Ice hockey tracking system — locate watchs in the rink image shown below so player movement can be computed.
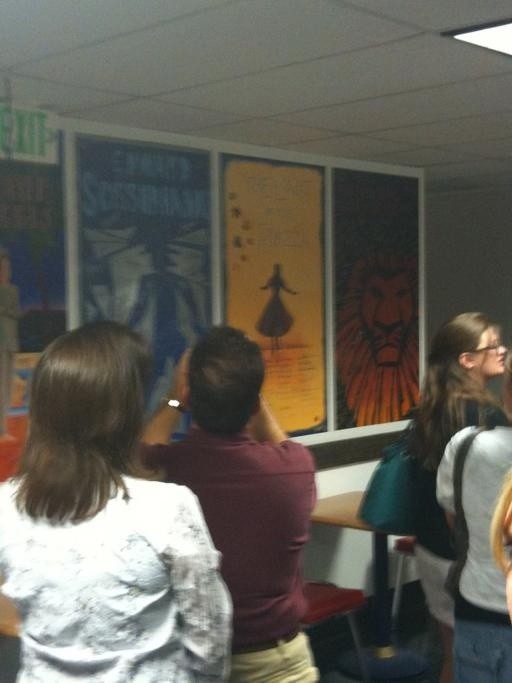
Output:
[161,394,188,413]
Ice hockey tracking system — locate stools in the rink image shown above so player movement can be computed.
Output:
[298,582,372,683]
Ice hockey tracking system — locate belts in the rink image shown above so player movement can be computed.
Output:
[231,625,300,654]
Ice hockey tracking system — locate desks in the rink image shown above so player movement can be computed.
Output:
[309,491,431,680]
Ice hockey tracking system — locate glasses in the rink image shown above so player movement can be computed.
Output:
[473,340,501,352]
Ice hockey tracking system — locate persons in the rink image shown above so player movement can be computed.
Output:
[435,344,512,682]
[133,326,325,682]
[1,319,234,683]
[0,247,48,443]
[254,260,299,355]
[404,312,511,682]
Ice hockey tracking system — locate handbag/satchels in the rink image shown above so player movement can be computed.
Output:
[356,428,422,535]
